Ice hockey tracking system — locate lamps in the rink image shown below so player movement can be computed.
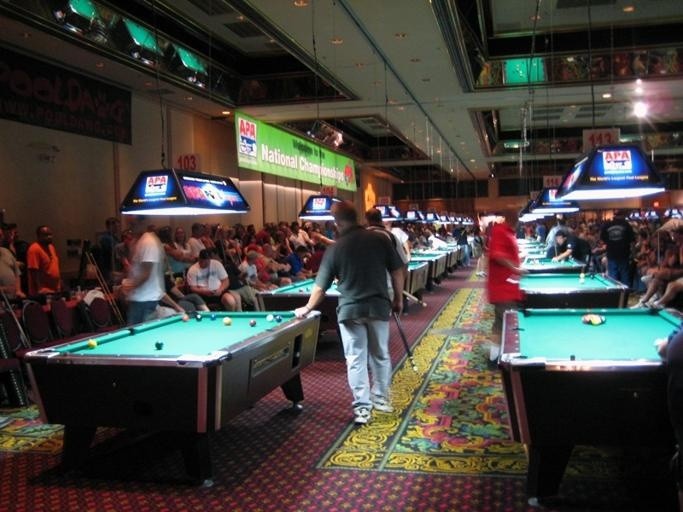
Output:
[662,206,682,220]
[451,216,477,226]
[369,56,405,222]
[442,138,451,225]
[118,0,251,216]
[427,123,440,224]
[293,1,348,221]
[553,2,667,202]
[644,208,660,221]
[625,209,642,221]
[529,56,581,216]
[516,200,544,222]
[407,98,427,224]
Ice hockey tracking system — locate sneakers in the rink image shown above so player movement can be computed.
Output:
[367,394,394,412]
[354,407,372,424]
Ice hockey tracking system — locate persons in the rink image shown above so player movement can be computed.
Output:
[26,225,62,303]
[189,222,206,258]
[156,278,212,318]
[0,222,10,248]
[274,219,338,287]
[114,214,165,326]
[157,226,200,282]
[225,222,274,312]
[486,208,530,371]
[186,250,236,312]
[543,210,683,312]
[6,223,26,264]
[146,224,159,238]
[174,227,198,272]
[79,217,122,272]
[657,314,683,386]
[517,221,545,242]
[119,230,133,269]
[293,200,404,426]
[406,221,448,250]
[0,246,26,304]
[472,230,487,278]
[210,223,223,248]
[457,225,470,268]
[202,222,215,252]
[390,221,411,287]
[365,208,404,311]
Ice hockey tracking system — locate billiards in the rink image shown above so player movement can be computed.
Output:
[580,272,586,284]
[182,314,190,322]
[590,274,595,279]
[196,314,202,320]
[306,287,311,292]
[276,315,282,322]
[541,261,545,266]
[582,313,606,326]
[87,339,96,347]
[299,288,303,292]
[224,317,231,325]
[155,341,164,349]
[210,314,216,320]
[249,320,256,326]
[267,315,273,322]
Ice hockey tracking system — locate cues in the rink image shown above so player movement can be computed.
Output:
[656,224,661,299]
[390,280,429,309]
[235,240,262,311]
[552,227,558,262]
[390,311,421,372]
[216,223,230,269]
[83,248,126,327]
